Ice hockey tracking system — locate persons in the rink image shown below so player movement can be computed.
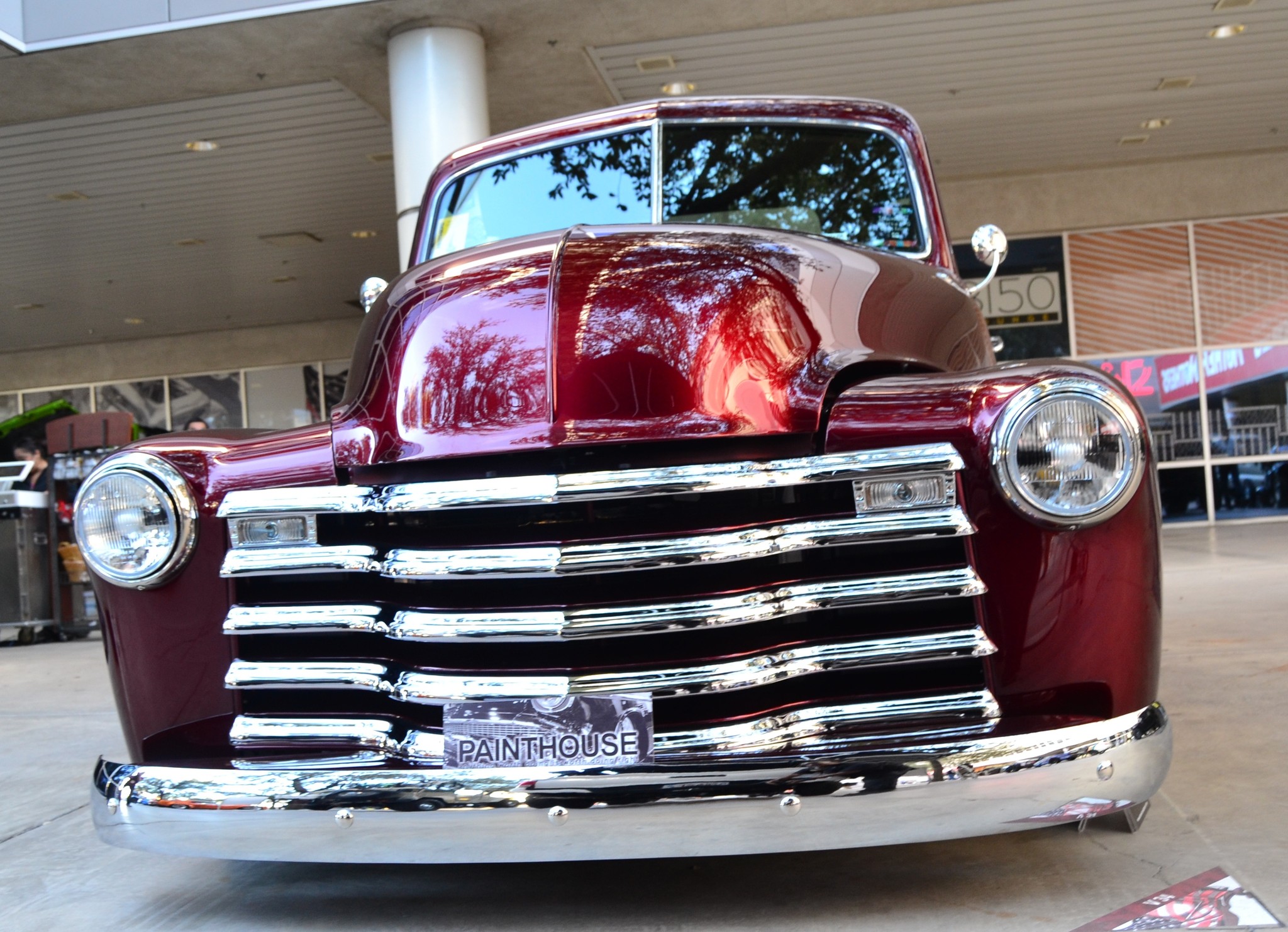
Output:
[1212,428,1247,511]
[8,438,56,494]
[184,418,210,433]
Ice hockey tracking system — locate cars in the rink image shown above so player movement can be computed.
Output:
[66,80,1169,868]
[1218,463,1267,503]
